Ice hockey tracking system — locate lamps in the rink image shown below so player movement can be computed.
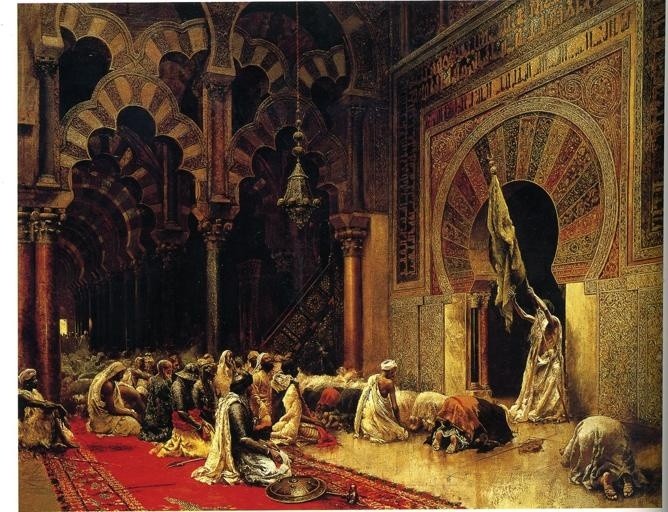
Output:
[277,2,322,230]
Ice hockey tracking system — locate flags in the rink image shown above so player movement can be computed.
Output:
[487,173,527,334]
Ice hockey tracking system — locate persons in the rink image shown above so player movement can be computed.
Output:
[508,286,569,423]
[560,414,651,501]
[17,329,511,493]
